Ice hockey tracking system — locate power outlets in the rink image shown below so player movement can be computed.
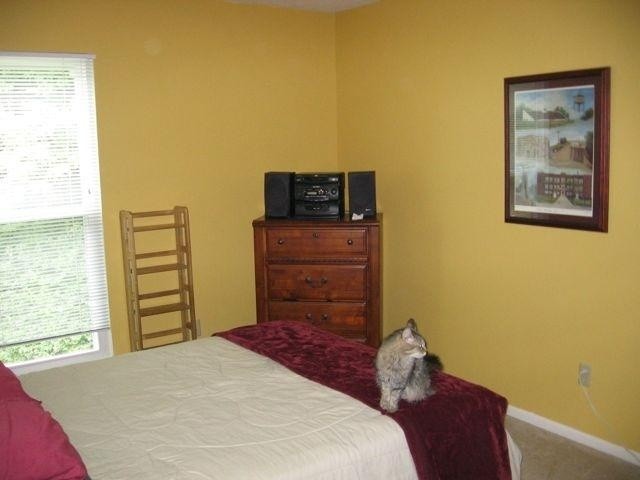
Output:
[576,361,593,388]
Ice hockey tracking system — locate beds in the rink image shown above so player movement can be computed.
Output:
[1,317,510,480]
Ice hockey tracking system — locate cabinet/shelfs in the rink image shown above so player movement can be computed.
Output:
[251,213,385,350]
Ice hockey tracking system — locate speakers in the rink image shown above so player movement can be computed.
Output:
[265,171,295,219]
[348,172,376,219]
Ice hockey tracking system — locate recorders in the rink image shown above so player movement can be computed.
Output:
[295,171,345,220]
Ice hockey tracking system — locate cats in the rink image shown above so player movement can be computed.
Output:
[373,317,443,415]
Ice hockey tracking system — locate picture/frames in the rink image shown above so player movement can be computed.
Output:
[502,66,612,234]
